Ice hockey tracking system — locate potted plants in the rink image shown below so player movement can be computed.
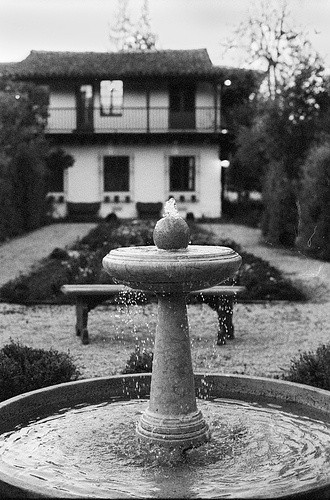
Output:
[169,195,174,200]
[114,195,120,203]
[48,195,55,204]
[59,195,64,203]
[125,195,131,203]
[191,194,197,203]
[179,196,186,202]
[104,196,110,202]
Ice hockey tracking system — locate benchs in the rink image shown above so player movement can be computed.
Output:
[59,283,248,346]
